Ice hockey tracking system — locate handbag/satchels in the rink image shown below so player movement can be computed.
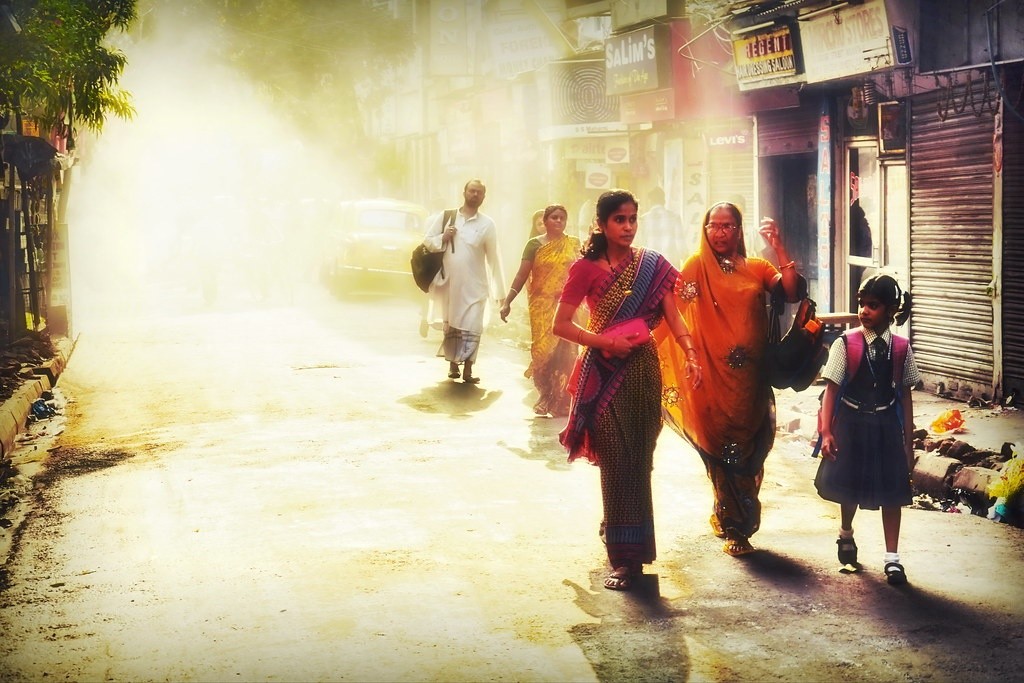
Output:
[597,317,649,359]
[411,209,457,293]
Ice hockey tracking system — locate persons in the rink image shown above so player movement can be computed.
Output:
[552,189,706,589]
[652,200,809,556]
[419,180,684,417]
[812,273,922,583]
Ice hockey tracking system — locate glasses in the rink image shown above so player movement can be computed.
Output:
[704,223,740,232]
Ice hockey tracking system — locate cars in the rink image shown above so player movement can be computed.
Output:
[315,198,431,316]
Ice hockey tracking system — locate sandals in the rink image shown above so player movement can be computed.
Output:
[710,513,727,538]
[604,570,642,590]
[723,537,754,557]
[598,520,606,544]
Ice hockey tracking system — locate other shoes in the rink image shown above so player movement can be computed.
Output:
[884,562,908,585]
[447,369,461,378]
[559,402,571,416]
[462,376,480,384]
[533,394,553,415]
[836,535,858,565]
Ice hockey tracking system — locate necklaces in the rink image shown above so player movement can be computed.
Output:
[604,247,635,295]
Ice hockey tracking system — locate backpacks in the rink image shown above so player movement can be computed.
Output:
[767,272,829,392]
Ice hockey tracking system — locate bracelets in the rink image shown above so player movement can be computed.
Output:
[778,261,795,271]
[685,348,696,356]
[510,288,518,295]
[675,334,691,342]
[578,329,583,347]
